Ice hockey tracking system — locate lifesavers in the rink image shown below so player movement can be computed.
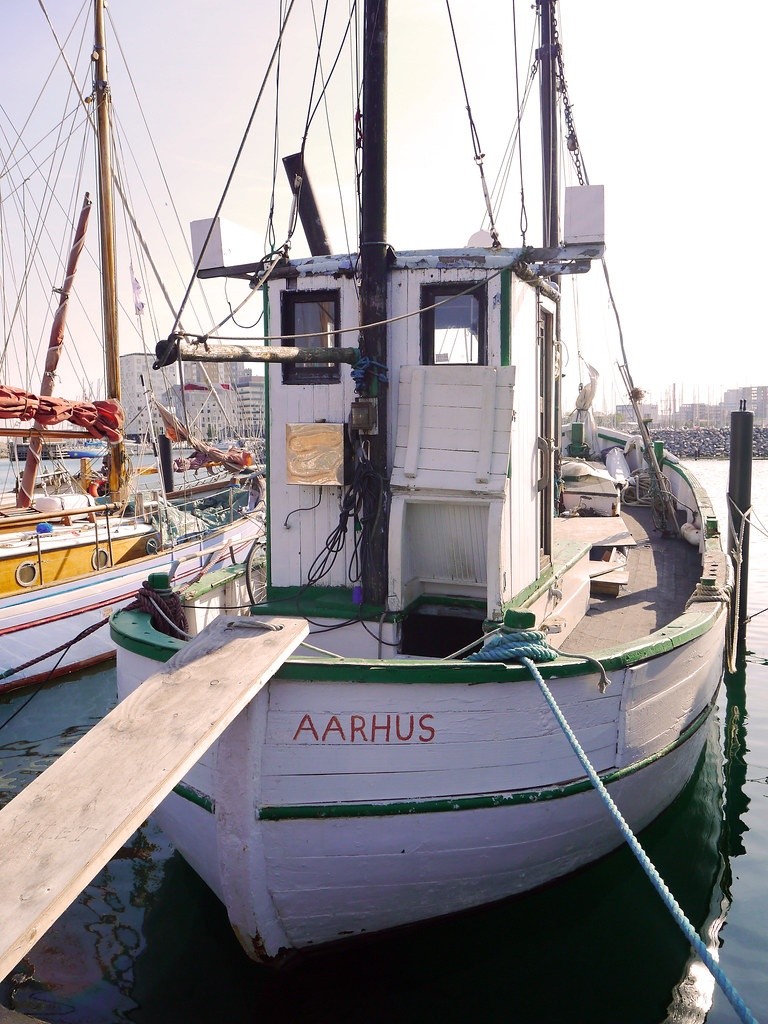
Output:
[90,479,110,497]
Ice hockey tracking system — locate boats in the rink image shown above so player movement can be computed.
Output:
[65,437,106,457]
[0,430,269,689]
[109,237,723,955]
